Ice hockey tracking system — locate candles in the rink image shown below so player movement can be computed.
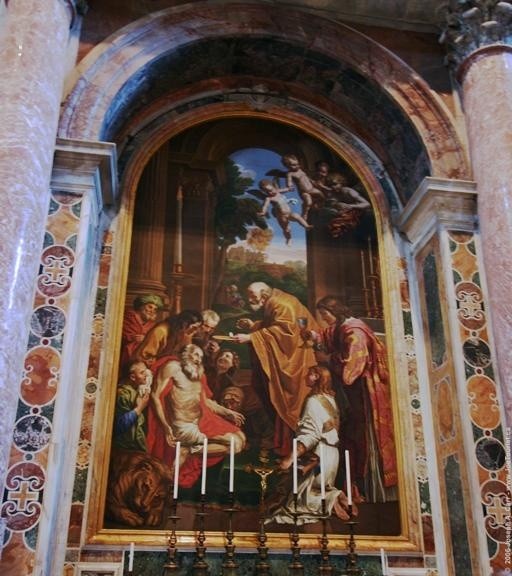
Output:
[201,438,208,495]
[173,441,181,499]
[380,548,386,576]
[320,447,325,499]
[292,439,298,493]
[128,541,134,571]
[229,437,235,492]
[345,450,352,505]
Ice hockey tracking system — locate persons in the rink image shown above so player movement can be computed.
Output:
[309,161,334,199]
[280,154,324,222]
[255,179,314,246]
[323,172,371,212]
